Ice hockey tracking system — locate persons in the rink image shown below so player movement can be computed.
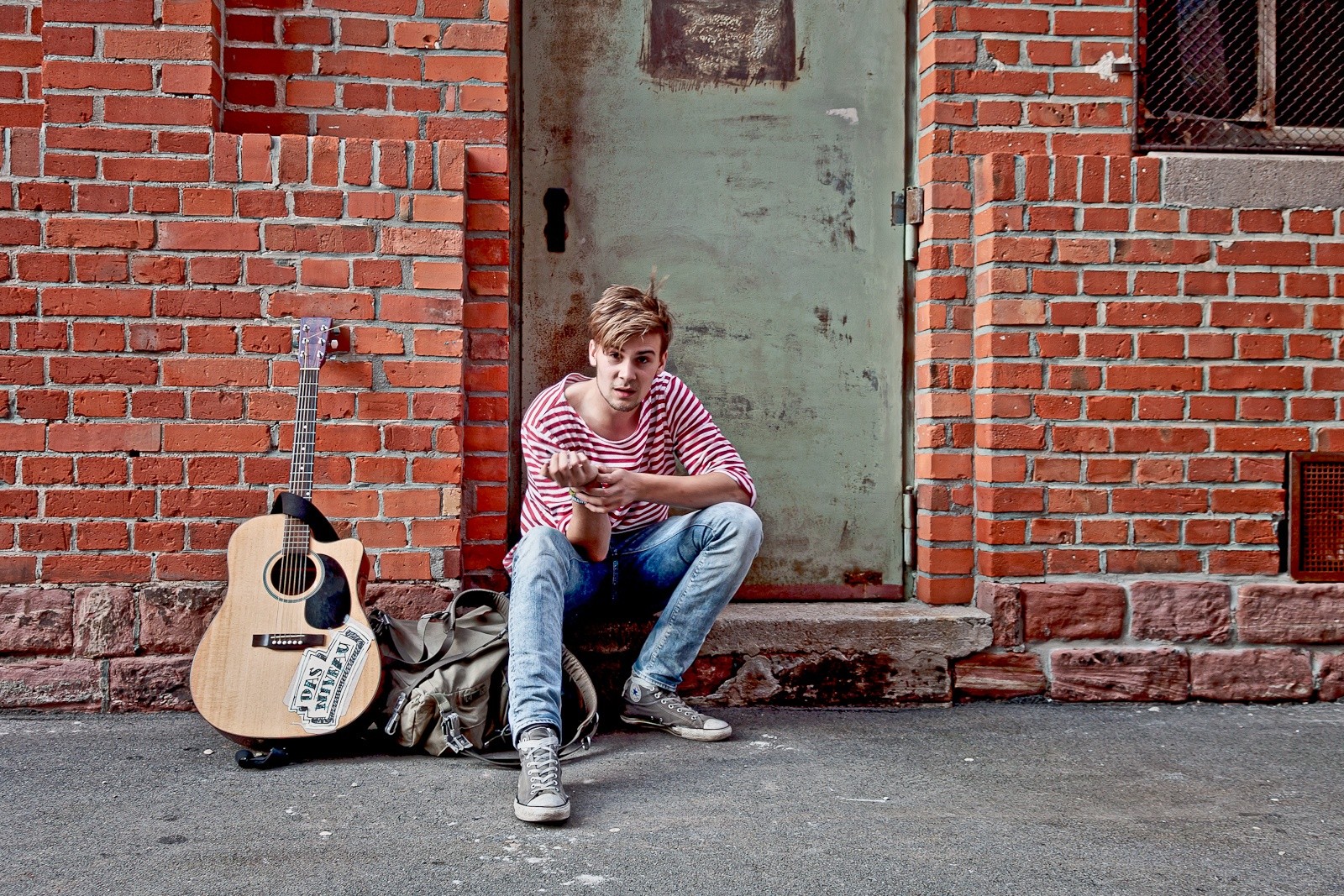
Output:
[501,265,764,823]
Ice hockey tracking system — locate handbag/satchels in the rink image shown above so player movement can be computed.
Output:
[364,592,515,759]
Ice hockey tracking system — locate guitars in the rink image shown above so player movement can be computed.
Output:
[189,314,385,749]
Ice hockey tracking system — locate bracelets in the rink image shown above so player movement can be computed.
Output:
[569,483,604,505]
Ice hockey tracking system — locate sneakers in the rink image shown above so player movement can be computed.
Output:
[514,727,571,821]
[619,677,732,741]
